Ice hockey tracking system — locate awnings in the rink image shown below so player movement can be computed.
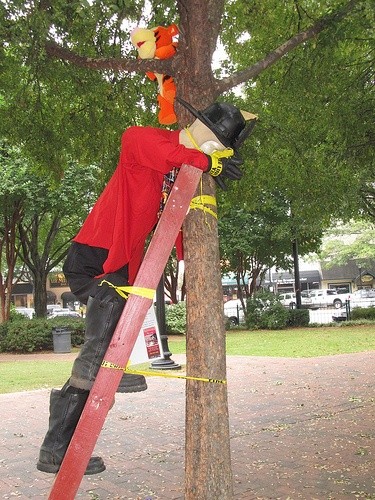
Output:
[46,291,56,300]
[4,284,33,296]
[61,292,75,301]
[222,272,249,285]
[264,270,320,284]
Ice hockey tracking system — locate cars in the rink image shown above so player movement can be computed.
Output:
[10,304,81,319]
[224,298,271,329]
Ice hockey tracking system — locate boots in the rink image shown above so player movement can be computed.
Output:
[69,296,148,393]
[38,390,105,474]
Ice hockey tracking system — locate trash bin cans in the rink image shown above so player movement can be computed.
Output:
[51,326,71,353]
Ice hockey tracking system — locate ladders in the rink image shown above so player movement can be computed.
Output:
[40,158,209,500]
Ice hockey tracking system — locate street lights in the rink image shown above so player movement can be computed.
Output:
[358,264,362,290]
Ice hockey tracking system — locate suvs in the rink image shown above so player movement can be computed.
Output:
[277,292,312,310]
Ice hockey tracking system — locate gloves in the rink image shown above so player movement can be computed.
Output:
[205,158,245,190]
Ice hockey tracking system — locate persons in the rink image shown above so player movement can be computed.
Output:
[37,98,261,475]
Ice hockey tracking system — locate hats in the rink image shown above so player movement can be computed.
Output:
[177,100,246,160]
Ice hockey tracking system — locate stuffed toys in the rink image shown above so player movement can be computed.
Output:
[131,25,179,125]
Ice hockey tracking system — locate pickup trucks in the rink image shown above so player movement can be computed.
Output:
[303,289,351,309]
